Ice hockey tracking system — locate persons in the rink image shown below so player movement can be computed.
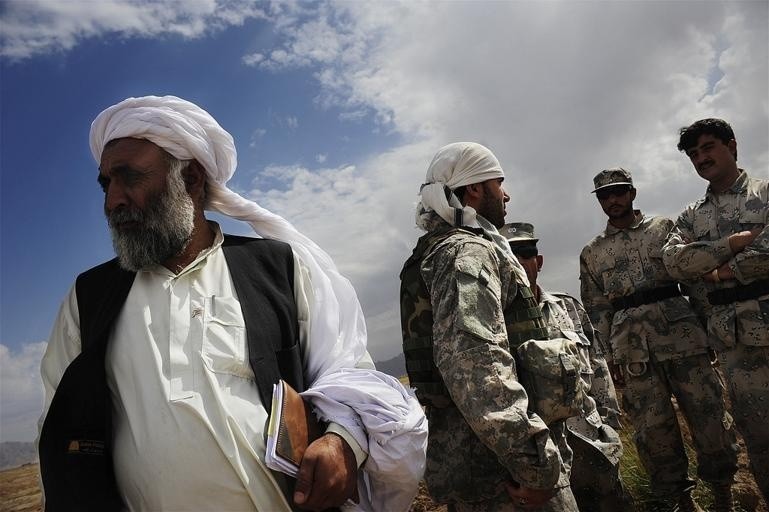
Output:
[580,168,743,512]
[661,119,769,506]
[398,141,582,512]
[35,95,430,512]
[500,223,634,512]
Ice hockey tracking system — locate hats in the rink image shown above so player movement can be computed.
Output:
[498,222,539,242]
[590,168,633,193]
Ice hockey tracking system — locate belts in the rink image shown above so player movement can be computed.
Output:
[706,279,768,305]
[610,283,682,313]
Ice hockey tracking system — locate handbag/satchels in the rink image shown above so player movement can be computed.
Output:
[518,337,597,425]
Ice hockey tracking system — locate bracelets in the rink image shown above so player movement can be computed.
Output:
[712,269,720,283]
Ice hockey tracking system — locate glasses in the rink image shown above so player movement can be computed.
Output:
[596,186,631,201]
[511,245,538,259]
